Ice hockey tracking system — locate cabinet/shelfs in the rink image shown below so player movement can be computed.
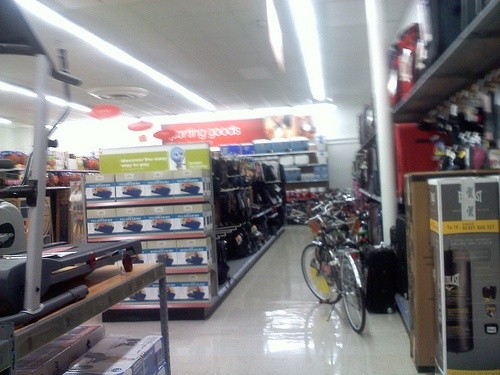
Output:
[242,151,330,201]
[357,135,383,202]
[393,0,500,336]
[84,142,285,323]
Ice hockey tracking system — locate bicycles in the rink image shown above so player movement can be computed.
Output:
[299,192,367,333]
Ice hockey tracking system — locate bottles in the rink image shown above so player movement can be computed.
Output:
[444,249,474,354]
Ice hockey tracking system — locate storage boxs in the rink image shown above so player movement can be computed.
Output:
[86,169,212,204]
[86,203,213,238]
[280,154,326,181]
[404,169,500,373]
[16,323,169,375]
[3,197,54,243]
[428,174,500,375]
[116,272,212,306]
[218,136,316,155]
[138,237,212,267]
